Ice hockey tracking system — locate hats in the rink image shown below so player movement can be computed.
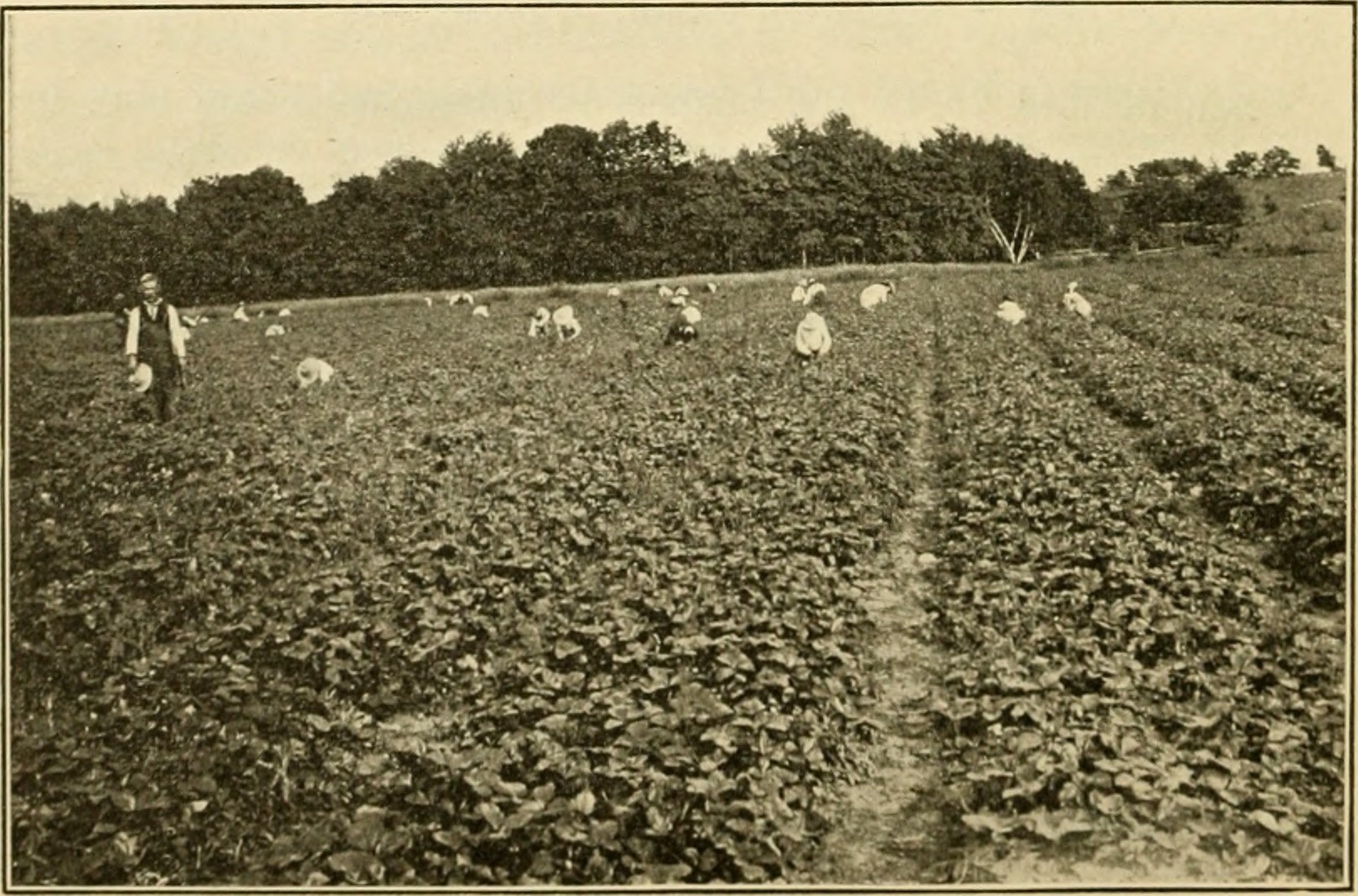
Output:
[798,278,808,286]
[885,279,896,296]
[531,307,550,327]
[265,324,286,336]
[809,278,815,282]
[238,300,245,305]
[802,312,820,328]
[297,356,318,382]
[279,308,291,318]
[682,305,701,323]
[554,305,573,325]
[128,362,152,393]
[609,283,614,286]
[182,326,191,339]
[707,282,717,293]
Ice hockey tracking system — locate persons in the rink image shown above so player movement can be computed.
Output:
[180,273,844,358]
[114,281,133,346]
[1062,281,1097,325]
[297,357,334,389]
[995,294,1026,326]
[858,279,897,312]
[123,270,187,419]
